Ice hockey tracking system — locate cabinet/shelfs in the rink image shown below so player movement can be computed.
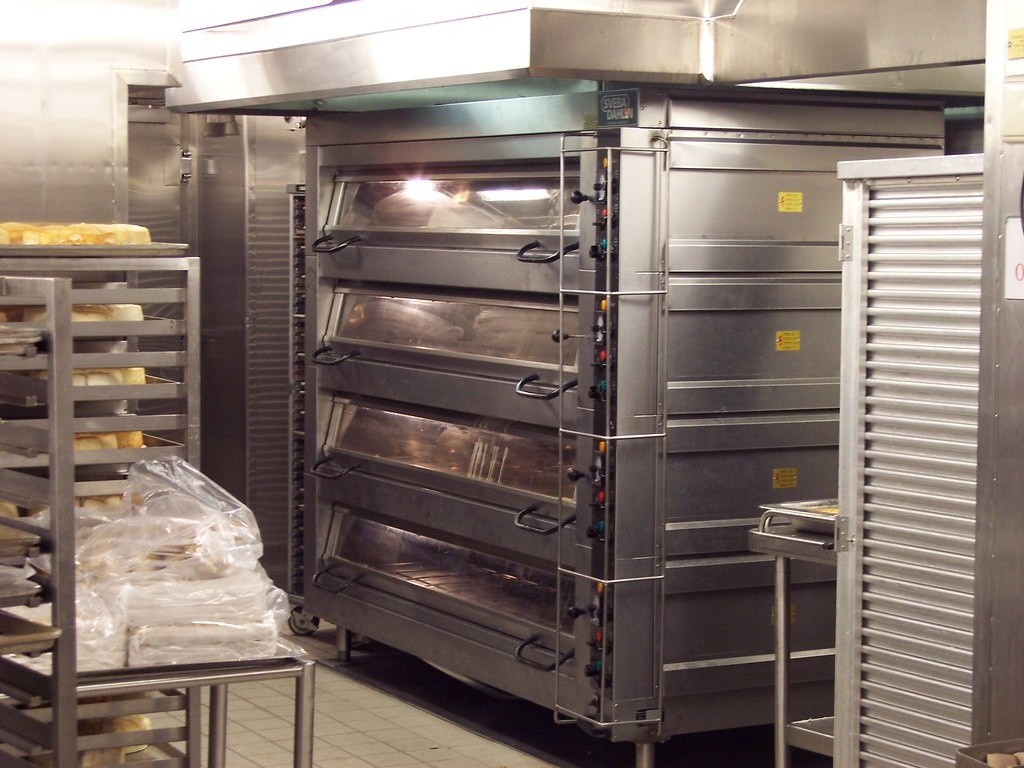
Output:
[0,255,200,768]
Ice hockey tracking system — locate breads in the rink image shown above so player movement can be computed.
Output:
[24,694,154,768]
[0,221,152,522]
